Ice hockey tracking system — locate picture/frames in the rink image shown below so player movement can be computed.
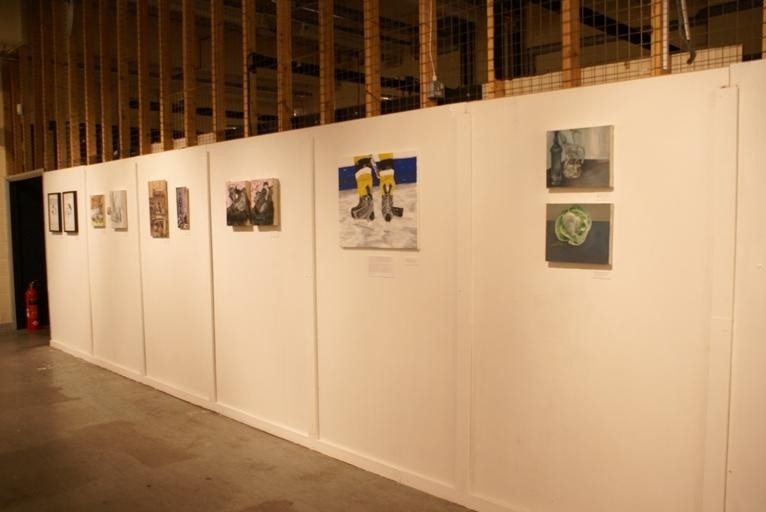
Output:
[46,191,78,233]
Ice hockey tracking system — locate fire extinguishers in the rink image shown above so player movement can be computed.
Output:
[24,280,44,332]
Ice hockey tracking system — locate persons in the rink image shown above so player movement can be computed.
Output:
[351,153,405,223]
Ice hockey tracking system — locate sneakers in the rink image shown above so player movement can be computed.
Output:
[351,196,375,219]
[382,194,393,221]
